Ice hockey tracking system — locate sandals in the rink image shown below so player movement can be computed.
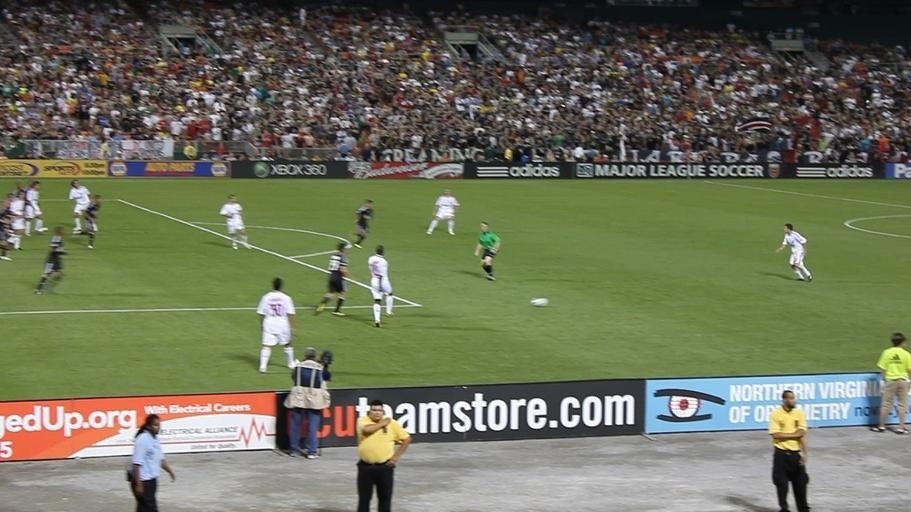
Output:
[290,448,321,460]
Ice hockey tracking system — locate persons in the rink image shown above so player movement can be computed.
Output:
[217,193,252,251]
[876,332,911,434]
[315,242,346,317]
[775,224,813,282]
[366,244,395,327]
[352,199,374,248]
[1,179,101,296]
[128,413,176,512]
[474,221,502,281]
[355,399,411,512]
[281,346,331,459]
[256,278,299,373]
[426,188,460,235]
[768,390,811,512]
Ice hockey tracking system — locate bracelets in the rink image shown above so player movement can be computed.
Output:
[389,458,395,465]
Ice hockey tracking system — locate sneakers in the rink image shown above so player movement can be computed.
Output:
[374,312,394,328]
[315,304,346,317]
[486,272,496,281]
[0,223,98,296]
[312,457,313,458]
[288,362,296,370]
[871,424,911,435]
[258,367,267,374]
[231,240,253,251]
[425,230,434,235]
[795,273,814,282]
[353,241,363,250]
[448,231,455,236]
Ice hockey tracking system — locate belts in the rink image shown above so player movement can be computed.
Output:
[774,446,801,454]
[360,459,390,466]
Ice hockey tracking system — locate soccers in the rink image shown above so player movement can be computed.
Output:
[531,299,549,307]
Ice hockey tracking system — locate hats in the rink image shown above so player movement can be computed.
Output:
[304,347,316,357]
